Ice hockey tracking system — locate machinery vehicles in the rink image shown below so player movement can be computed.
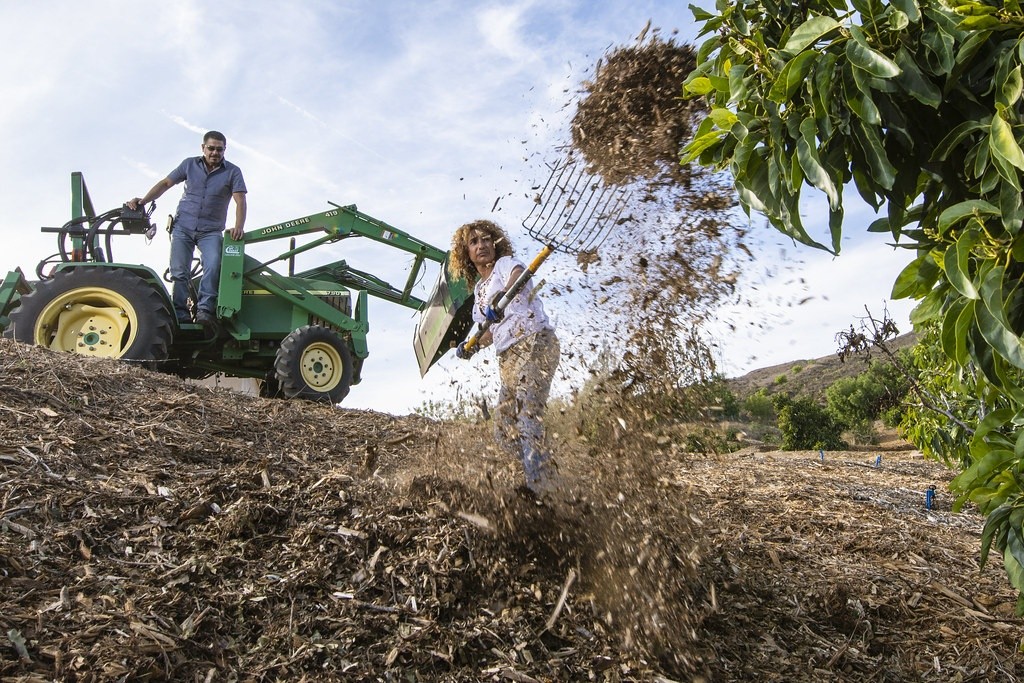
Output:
[0,171,479,406]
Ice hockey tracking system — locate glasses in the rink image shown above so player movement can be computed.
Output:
[204,145,225,151]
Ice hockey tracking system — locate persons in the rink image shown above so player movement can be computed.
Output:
[451,216,565,502]
[123,130,247,323]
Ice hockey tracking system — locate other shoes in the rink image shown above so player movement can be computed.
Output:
[175,308,193,324]
[196,310,213,325]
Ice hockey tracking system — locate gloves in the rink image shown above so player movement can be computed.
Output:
[456,341,480,360]
[486,307,505,324]
